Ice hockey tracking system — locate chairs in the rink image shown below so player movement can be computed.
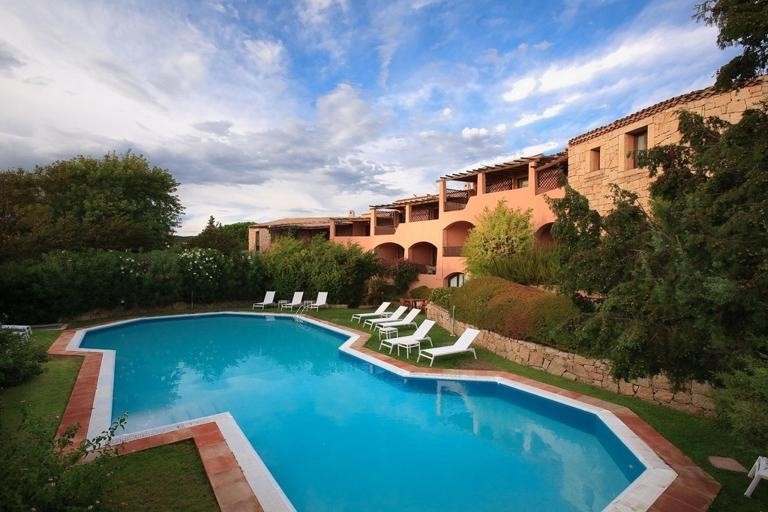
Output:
[745,455,768,498]
[252,290,329,312]
[350,300,482,367]
[0,323,33,343]
